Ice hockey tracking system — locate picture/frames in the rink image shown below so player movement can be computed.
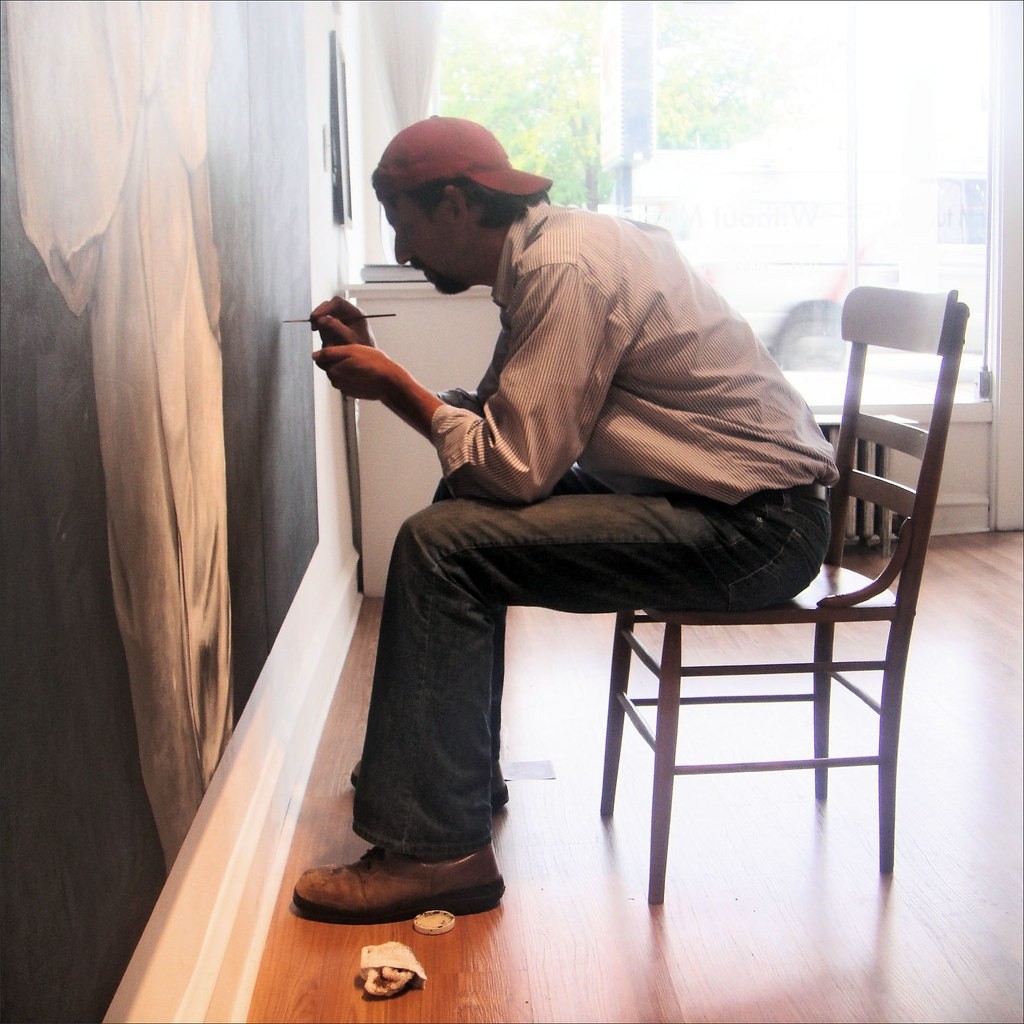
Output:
[329,30,353,230]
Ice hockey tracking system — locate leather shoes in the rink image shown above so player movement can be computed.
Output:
[350,759,509,813]
[294,844,505,923]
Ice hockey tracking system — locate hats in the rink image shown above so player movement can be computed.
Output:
[371,114,554,207]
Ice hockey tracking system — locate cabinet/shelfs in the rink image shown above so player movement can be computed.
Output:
[346,284,502,596]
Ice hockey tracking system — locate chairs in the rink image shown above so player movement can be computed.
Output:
[600,287,970,905]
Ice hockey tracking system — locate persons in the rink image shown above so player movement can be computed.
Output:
[290,116,839,922]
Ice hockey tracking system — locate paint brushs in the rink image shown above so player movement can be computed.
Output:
[283,313,396,323]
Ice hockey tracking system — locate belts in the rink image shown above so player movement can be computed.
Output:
[789,480,830,504]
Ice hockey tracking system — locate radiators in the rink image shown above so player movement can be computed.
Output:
[811,412,918,557]
